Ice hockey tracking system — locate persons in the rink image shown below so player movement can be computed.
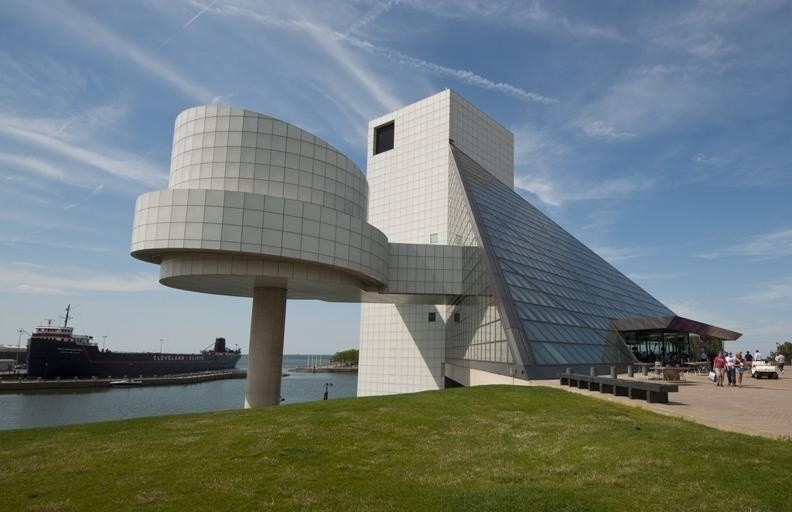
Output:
[733,352,744,388]
[777,354,785,375]
[745,351,752,361]
[713,351,727,386]
[699,348,728,371]
[754,349,762,361]
[725,352,736,385]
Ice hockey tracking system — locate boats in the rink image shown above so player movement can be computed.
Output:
[109,378,143,389]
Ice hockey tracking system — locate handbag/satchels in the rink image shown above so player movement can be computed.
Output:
[708,372,716,382]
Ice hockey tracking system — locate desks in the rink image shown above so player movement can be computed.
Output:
[633,360,712,381]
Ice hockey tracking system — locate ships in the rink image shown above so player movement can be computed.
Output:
[25,302,244,378]
